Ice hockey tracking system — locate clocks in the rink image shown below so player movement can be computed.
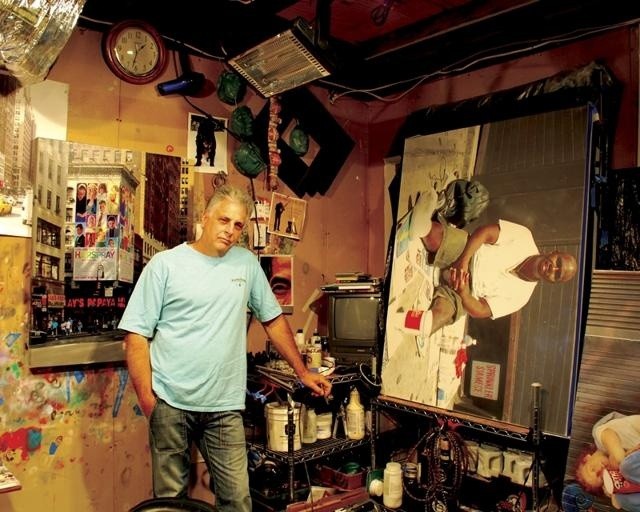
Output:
[101,18,167,85]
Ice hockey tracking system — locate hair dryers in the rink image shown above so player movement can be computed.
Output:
[157,40,205,97]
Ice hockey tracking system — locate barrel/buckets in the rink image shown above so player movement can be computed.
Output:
[264,400,306,454]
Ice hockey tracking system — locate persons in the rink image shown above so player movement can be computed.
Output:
[274,202,285,232]
[48,315,119,336]
[413,187,576,336]
[268,257,291,305]
[66,183,134,253]
[574,410,640,512]
[117,183,332,512]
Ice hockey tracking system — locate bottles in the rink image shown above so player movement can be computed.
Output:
[311,328,329,352]
[346,387,365,439]
[383,462,403,508]
[294,327,306,352]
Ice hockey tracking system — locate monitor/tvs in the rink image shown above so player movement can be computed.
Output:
[327,292,382,354]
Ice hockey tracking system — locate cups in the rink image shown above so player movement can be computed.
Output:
[305,346,321,369]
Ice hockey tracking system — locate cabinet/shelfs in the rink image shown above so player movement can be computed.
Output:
[246,348,404,512]
